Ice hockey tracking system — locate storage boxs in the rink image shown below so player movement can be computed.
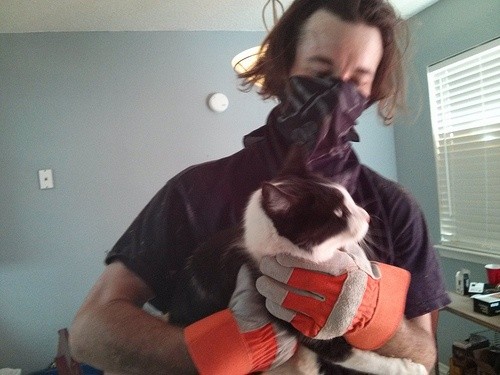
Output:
[470,294,500,317]
[448,333,500,375]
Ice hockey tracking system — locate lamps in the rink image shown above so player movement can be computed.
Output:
[230,0,290,88]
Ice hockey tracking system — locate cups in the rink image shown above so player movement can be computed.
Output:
[485,264,500,289]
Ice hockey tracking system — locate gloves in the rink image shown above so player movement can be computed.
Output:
[257,249,410,347]
[184,265,297,375]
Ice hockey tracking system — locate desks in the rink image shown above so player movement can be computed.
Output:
[444,290,500,333]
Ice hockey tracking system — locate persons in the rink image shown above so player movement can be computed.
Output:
[65,0,449,375]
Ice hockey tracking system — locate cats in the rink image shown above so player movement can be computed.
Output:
[168,128,428,375]
